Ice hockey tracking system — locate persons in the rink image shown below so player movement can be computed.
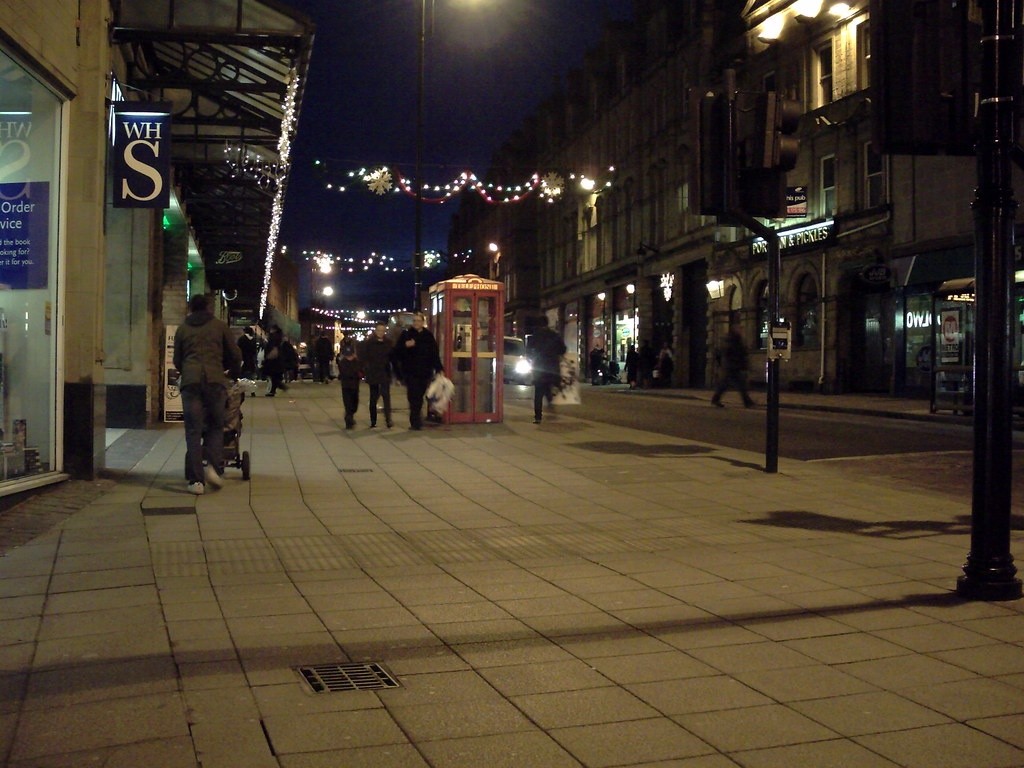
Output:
[307,332,336,384]
[361,323,401,428]
[623,343,673,390]
[589,344,608,386]
[336,330,360,429]
[711,316,757,411]
[400,314,442,430]
[527,315,566,424]
[173,295,243,494]
[264,325,299,397]
[238,327,256,381]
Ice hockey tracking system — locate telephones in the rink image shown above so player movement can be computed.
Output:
[455,324,471,353]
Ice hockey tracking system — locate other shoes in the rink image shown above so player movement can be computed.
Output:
[712,400,725,407]
[265,393,276,397]
[188,479,205,495]
[533,418,540,424]
[408,424,418,431]
[204,465,224,488]
[371,414,376,426]
[747,402,757,407]
[386,420,394,428]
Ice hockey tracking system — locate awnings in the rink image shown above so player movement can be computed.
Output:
[107,1,316,319]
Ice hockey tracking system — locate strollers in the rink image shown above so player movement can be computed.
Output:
[176,371,250,481]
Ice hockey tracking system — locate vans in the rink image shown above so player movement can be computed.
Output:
[503,337,527,385]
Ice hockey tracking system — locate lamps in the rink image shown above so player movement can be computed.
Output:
[636,241,666,258]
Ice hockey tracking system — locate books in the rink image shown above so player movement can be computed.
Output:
[1,443,13,446]
[22,447,39,450]
